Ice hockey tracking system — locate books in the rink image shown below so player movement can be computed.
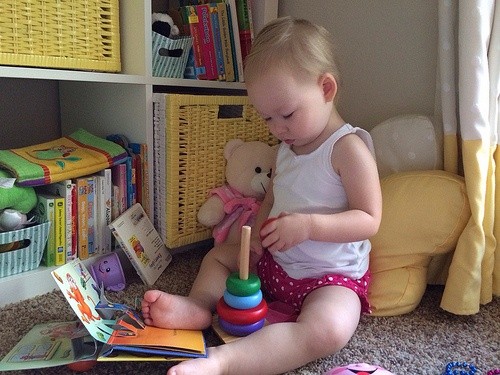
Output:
[37,143,149,266]
[108,203,172,285]
[165,1,254,83]
[0,257,208,371]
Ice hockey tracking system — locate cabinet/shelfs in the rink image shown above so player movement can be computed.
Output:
[0,1,279,309]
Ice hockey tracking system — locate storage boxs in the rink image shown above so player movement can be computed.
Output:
[0,214,51,278]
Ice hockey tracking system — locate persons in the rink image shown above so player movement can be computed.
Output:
[141,16,383,375]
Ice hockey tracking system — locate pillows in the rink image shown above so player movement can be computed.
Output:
[369,112,471,317]
